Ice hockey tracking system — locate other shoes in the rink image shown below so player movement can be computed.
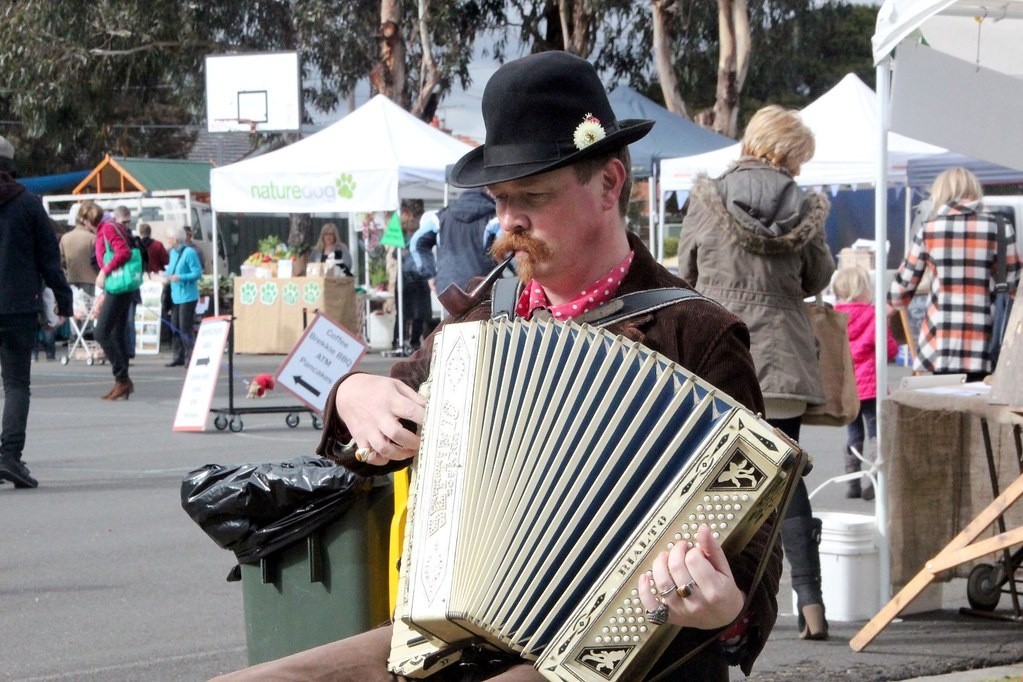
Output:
[0,457,39,487]
[165,359,185,367]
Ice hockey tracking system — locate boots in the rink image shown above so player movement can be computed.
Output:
[781,517,829,637]
[843,451,861,498]
[863,438,877,499]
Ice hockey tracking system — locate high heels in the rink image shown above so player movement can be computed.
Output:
[101,379,133,400]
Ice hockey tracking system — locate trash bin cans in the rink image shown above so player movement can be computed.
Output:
[362,290,397,352]
[240,454,395,667]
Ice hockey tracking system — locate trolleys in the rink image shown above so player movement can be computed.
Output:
[899,381,1023,624]
[208,308,329,433]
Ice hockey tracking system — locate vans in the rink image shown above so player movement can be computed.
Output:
[66,199,239,279]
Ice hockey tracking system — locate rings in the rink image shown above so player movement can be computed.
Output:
[677,580,696,597]
[658,585,676,597]
[355,447,374,462]
[645,603,669,625]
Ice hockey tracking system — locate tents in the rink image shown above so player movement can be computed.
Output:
[872,0,1022,601]
[660,72,1022,352]
[444,85,737,259]
[210,95,475,352]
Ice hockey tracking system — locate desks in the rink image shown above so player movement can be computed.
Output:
[231,276,357,355]
[846,387,1023,653]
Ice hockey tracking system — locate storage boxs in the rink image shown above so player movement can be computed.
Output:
[240,255,332,276]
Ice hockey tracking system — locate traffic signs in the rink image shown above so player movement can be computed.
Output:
[172,315,232,433]
[273,312,368,419]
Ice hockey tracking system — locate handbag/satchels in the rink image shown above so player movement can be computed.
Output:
[988,286,1010,359]
[161,284,173,308]
[102,224,143,294]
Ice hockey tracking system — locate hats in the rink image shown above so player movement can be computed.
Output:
[448,50,655,188]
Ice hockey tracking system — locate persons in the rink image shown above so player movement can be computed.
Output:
[409,185,502,322]
[833,266,898,500]
[679,105,836,637]
[0,137,74,488]
[206,50,784,682]
[32,202,205,400]
[307,224,353,270]
[887,168,1023,383]
[392,219,427,352]
